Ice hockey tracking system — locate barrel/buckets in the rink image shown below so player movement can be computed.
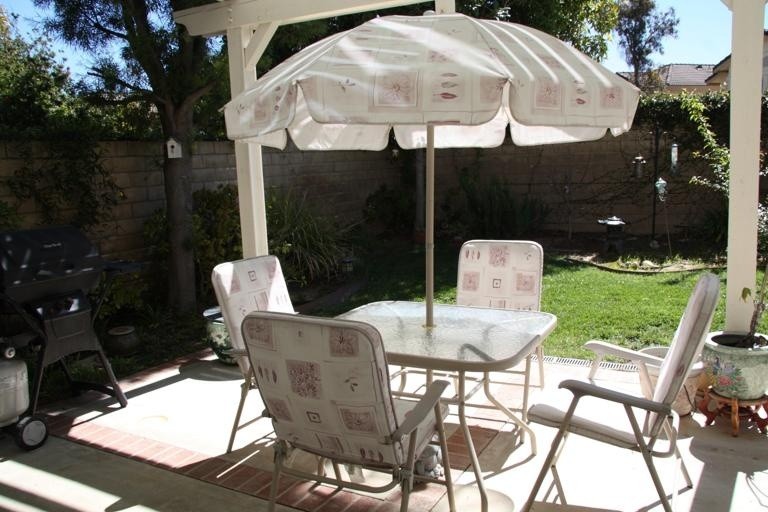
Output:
[632,347,703,417]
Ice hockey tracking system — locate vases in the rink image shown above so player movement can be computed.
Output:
[206,316,238,365]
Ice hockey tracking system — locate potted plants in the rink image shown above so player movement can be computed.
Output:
[702,249,768,401]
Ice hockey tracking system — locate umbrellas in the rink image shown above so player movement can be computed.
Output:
[218,7,645,392]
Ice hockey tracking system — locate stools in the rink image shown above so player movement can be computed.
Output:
[698,384,768,438]
